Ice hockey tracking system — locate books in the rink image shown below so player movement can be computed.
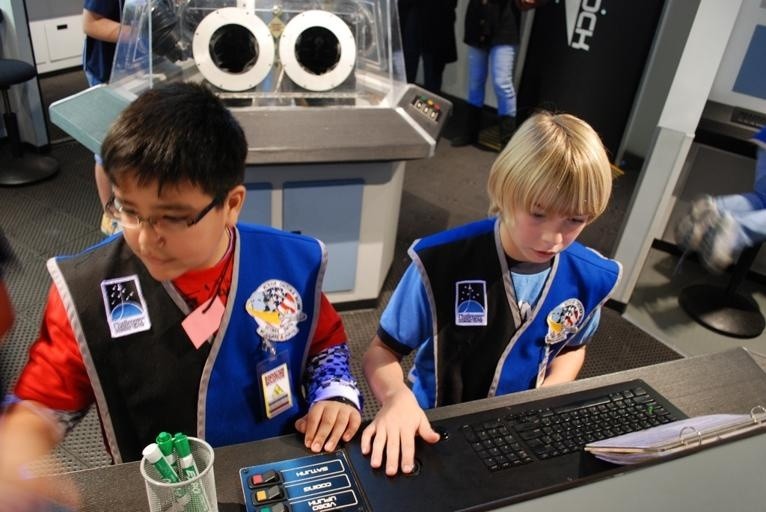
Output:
[584,410,766,465]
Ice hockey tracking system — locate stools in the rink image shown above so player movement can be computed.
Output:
[0,57,57,187]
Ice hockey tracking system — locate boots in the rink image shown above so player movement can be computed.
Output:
[451,102,483,146]
[496,114,515,152]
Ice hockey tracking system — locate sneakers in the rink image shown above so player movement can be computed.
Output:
[674,195,735,267]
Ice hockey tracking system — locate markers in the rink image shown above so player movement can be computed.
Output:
[141,431,212,512]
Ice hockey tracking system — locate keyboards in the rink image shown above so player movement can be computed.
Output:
[457,382,679,482]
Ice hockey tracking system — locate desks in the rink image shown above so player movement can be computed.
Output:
[17,344,764,511]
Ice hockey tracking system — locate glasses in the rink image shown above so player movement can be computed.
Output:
[104,193,228,237]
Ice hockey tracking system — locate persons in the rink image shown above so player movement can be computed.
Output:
[81,1,184,241]
[675,127,766,273]
[395,0,458,97]
[358,108,625,480]
[452,1,536,152]
[0,82,365,511]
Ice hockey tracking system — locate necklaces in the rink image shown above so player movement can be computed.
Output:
[161,223,239,314]
